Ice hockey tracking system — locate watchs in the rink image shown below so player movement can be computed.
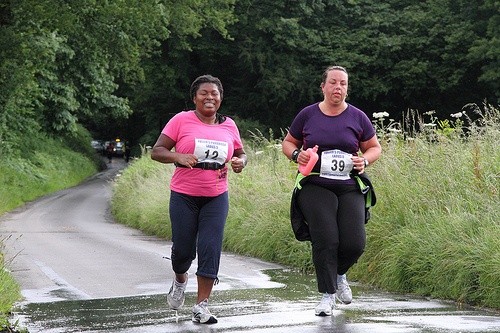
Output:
[292,149,300,163]
[365,159,369,167]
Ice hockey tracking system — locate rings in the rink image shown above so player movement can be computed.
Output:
[237,167,238,170]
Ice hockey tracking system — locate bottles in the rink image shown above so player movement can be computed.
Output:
[297,144,319,177]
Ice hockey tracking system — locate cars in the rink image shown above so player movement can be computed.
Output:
[91,139,125,156]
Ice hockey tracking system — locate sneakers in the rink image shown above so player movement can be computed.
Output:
[315,293,335,317]
[167,272,189,310]
[336,274,353,304]
[192,298,217,324]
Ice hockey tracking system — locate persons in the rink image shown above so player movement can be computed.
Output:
[282,66,381,316]
[107,143,114,163]
[151,74,247,324]
[125,138,131,163]
